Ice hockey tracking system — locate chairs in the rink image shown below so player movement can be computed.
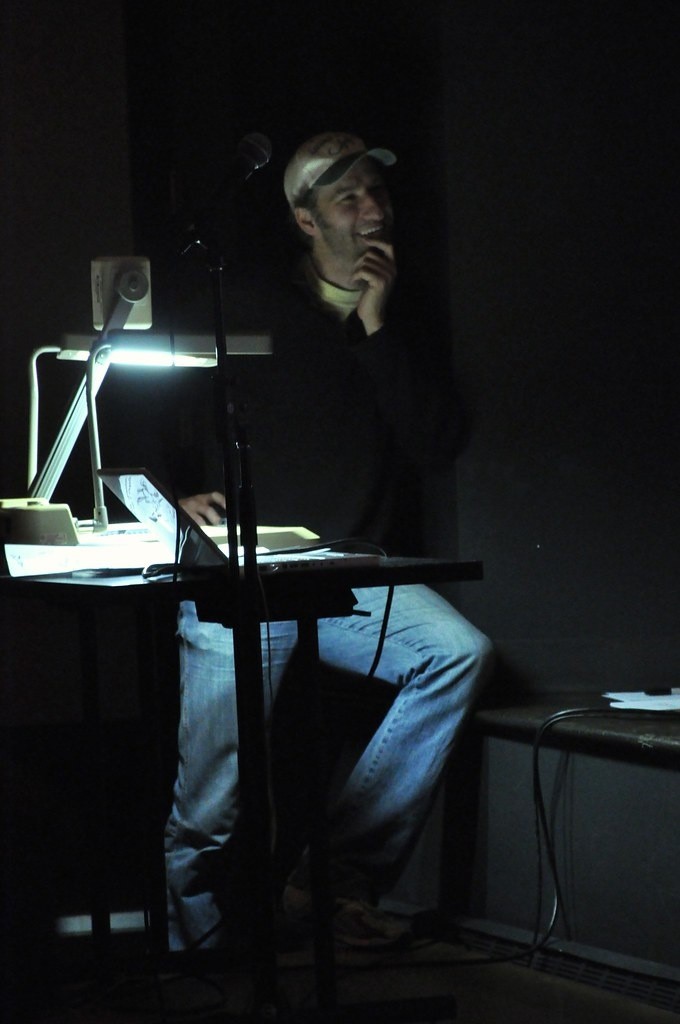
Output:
[140,600,503,950]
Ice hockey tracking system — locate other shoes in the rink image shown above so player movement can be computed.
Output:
[280,874,404,943]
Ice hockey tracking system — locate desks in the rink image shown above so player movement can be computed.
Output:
[0,550,482,1024]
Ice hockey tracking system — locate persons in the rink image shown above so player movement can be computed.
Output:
[162,127,494,964]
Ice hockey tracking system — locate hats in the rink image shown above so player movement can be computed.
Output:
[284,129,399,206]
